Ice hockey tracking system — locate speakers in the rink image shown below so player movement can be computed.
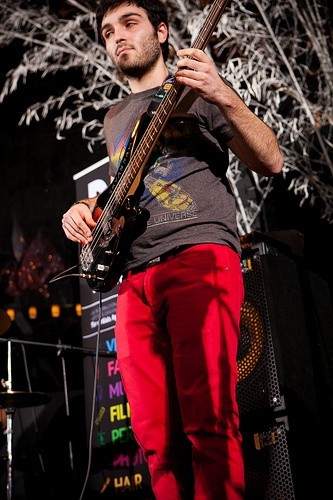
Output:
[234,249,328,500]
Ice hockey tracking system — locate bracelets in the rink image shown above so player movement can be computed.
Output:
[71,201,91,210]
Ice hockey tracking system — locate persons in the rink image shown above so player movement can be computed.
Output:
[62,0,285,499]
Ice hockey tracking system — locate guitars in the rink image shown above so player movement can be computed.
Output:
[78,0,227,293]
